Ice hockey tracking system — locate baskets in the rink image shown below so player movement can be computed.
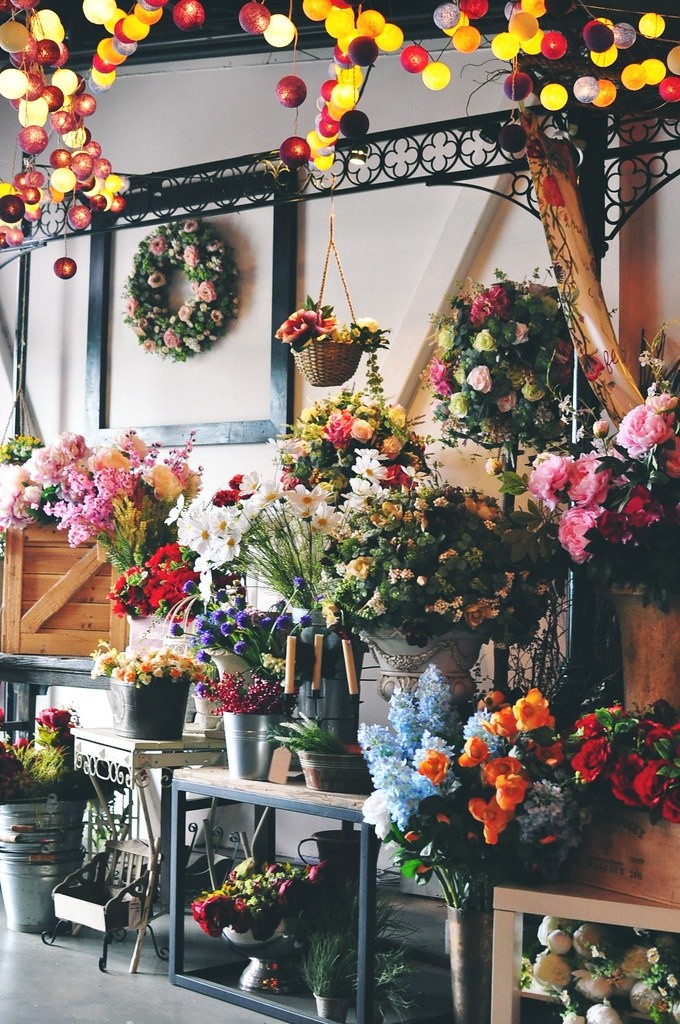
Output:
[290,338,363,387]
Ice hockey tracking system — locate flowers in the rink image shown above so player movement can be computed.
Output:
[0,212,680,1024]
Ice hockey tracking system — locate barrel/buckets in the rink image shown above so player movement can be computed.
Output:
[222,711,286,783]
[0,793,89,934]
[297,828,383,936]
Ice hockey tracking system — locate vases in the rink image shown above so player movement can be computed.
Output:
[356,619,483,710]
[221,910,311,995]
[210,655,252,690]
[313,831,381,892]
[292,609,361,750]
[295,747,372,794]
[223,711,288,781]
[607,579,680,715]
[109,679,191,741]
[446,905,493,1024]
[192,696,221,729]
[127,616,197,658]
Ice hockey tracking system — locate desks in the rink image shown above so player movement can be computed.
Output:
[490,885,680,1024]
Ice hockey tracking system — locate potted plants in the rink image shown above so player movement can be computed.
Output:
[285,873,421,1023]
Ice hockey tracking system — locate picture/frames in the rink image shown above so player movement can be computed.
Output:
[83,149,296,446]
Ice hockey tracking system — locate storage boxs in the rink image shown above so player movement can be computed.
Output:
[571,806,680,908]
[1,519,127,659]
